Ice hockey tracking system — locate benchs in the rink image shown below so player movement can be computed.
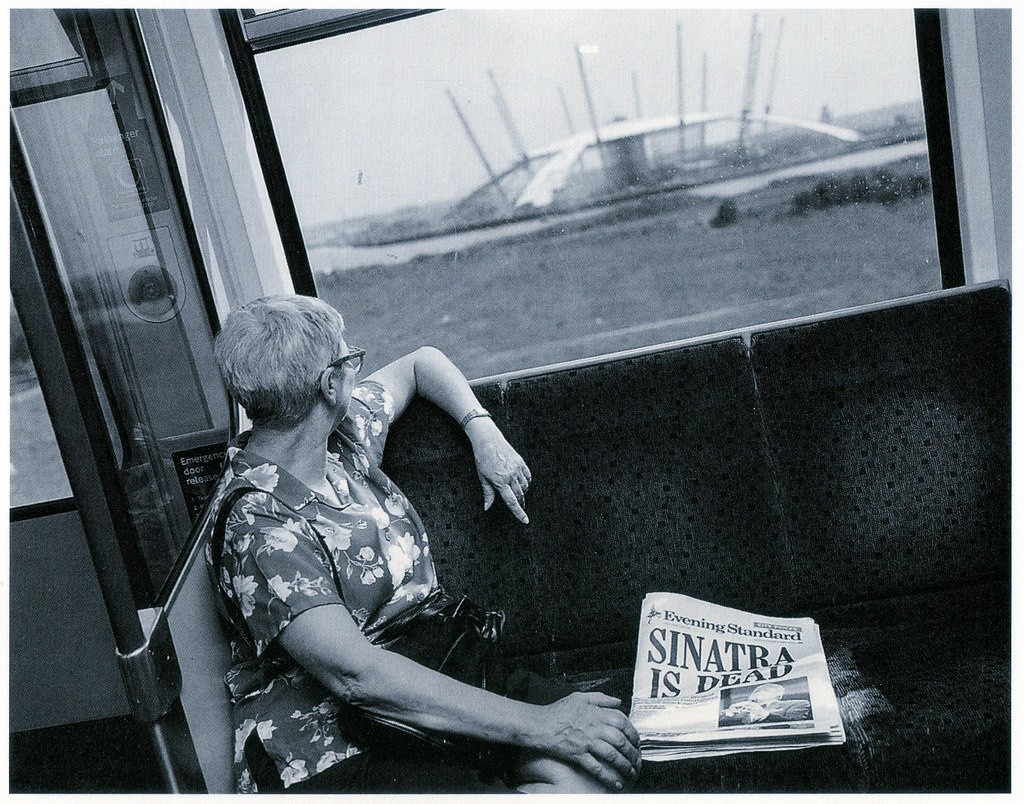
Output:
[125,279,1011,793]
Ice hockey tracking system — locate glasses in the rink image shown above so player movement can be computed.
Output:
[318,346,365,383]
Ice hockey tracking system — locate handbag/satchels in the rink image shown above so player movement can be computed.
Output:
[336,591,505,778]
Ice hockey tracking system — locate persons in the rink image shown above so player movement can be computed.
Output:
[211,295,644,794]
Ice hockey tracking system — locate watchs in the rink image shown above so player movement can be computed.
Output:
[459,406,492,431]
